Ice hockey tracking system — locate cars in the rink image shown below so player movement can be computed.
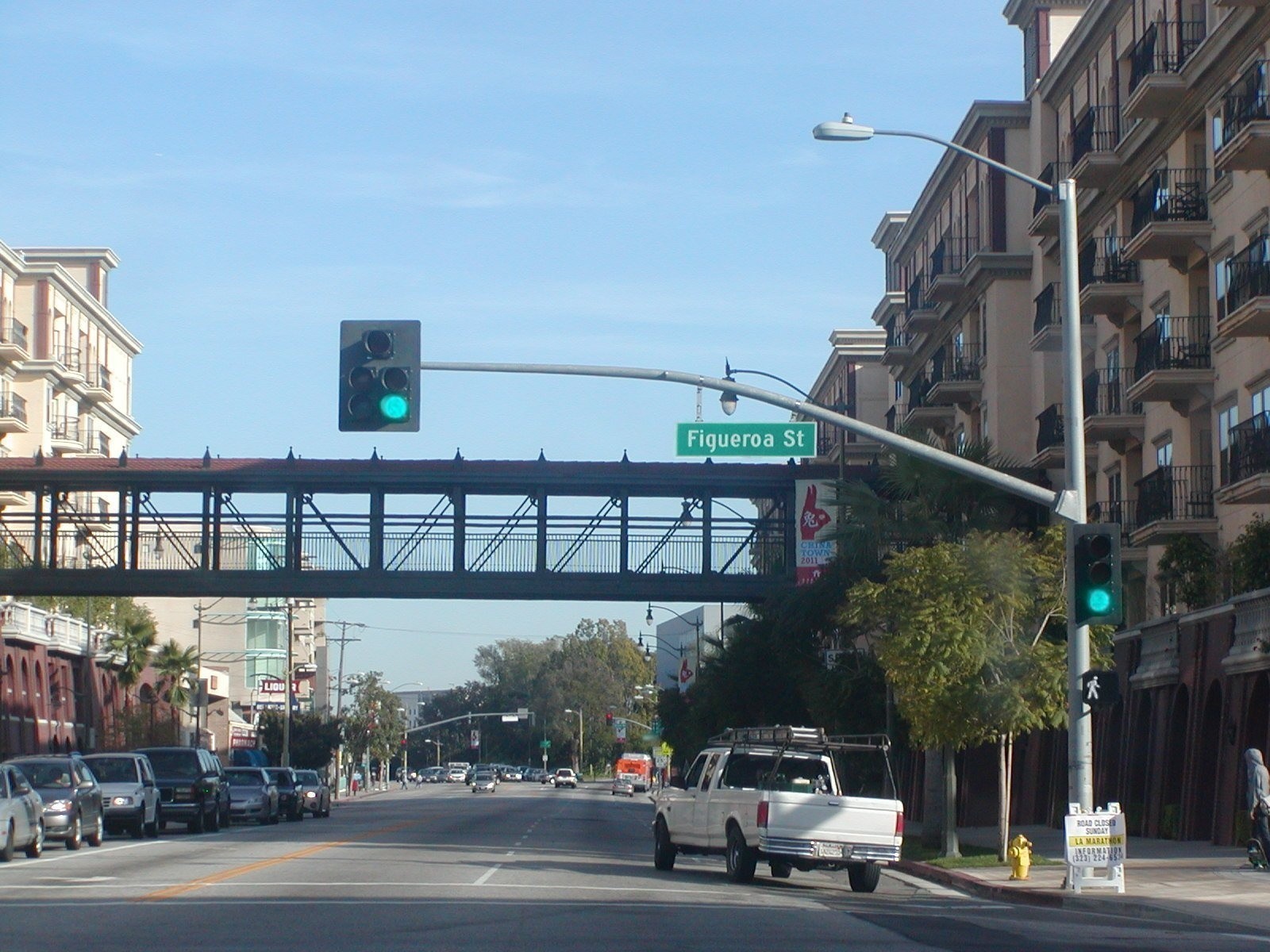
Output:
[612,778,635,798]
[396,762,578,793]
[0,763,46,863]
[223,766,331,825]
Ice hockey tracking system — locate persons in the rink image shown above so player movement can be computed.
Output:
[43,767,72,788]
[351,769,423,796]
[1245,748,1270,872]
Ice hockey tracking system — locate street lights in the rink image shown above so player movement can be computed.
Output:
[424,739,444,766]
[193,597,259,748]
[679,497,768,727]
[659,561,726,732]
[81,528,166,753]
[636,633,687,695]
[335,672,365,800]
[645,602,704,685]
[564,709,584,782]
[326,678,360,723]
[281,663,318,767]
[718,357,853,800]
[813,111,1096,889]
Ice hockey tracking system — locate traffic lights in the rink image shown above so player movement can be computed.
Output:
[1073,522,1124,628]
[338,317,421,433]
[606,713,613,726]
[1081,669,1120,705]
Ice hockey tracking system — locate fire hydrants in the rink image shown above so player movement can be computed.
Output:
[1006,834,1033,882]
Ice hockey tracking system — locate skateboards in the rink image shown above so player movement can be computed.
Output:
[1246,838,1270,871]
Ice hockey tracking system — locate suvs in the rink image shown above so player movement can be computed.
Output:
[75,752,162,839]
[209,750,232,830]
[2,751,105,851]
[121,746,220,835]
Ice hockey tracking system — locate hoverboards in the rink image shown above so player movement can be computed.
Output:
[1245,811,1270,871]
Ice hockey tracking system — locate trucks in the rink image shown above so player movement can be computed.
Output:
[616,753,653,793]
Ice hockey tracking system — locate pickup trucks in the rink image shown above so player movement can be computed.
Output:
[652,724,905,893]
